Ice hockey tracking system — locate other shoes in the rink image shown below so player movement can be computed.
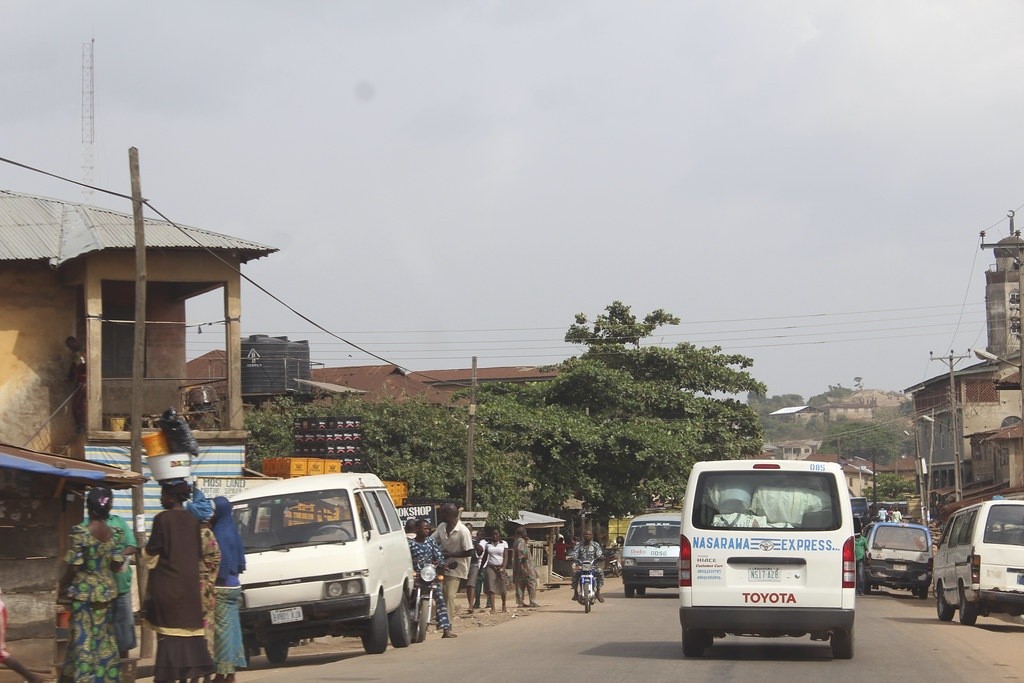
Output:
[595,594,604,602]
[473,605,480,608]
[572,592,578,600]
[442,633,457,638]
[530,604,540,607]
[518,604,528,608]
[485,605,492,608]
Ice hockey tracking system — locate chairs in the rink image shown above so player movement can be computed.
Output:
[319,519,364,536]
[241,530,280,546]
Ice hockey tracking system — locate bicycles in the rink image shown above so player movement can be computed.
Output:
[604,545,622,578]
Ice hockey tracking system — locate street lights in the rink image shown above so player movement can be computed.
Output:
[971,347,1024,446]
[922,415,962,501]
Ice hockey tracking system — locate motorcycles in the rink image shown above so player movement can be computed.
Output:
[410,560,457,643]
[565,552,604,613]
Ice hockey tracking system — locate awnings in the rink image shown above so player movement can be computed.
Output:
[0,444,147,487]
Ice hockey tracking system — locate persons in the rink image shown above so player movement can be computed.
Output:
[55,486,125,683]
[553,537,573,561]
[186,499,221,683]
[566,530,604,602]
[513,527,540,608]
[79,488,137,673]
[405,502,491,638]
[855,528,869,595]
[877,504,903,522]
[138,478,213,683]
[208,496,244,683]
[478,528,508,613]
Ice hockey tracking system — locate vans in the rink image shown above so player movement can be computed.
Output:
[932,500,1024,625]
[679,459,855,661]
[216,470,415,665]
[850,497,869,520]
[859,521,933,598]
[621,513,681,596]
